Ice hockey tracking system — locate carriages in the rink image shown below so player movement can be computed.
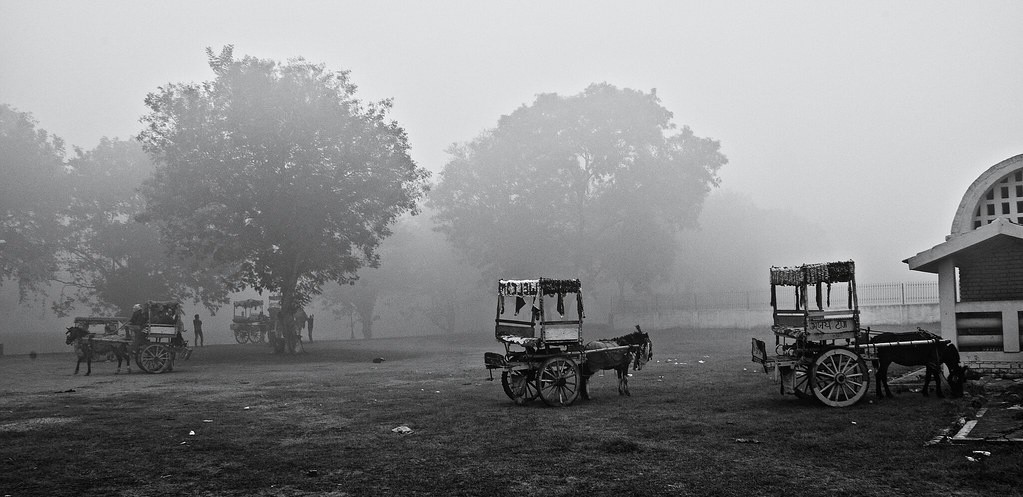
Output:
[230,299,283,346]
[750,259,968,410]
[269,295,308,345]
[485,276,653,409]
[63,300,193,376]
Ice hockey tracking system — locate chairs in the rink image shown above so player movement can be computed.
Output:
[494,317,583,346]
[776,307,860,341]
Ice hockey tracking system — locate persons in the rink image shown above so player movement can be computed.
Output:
[193,314,204,346]
[124,304,145,339]
[307,313,315,343]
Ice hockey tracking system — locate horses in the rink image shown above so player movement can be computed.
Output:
[65,326,131,377]
[574,332,652,400]
[869,331,968,401]
